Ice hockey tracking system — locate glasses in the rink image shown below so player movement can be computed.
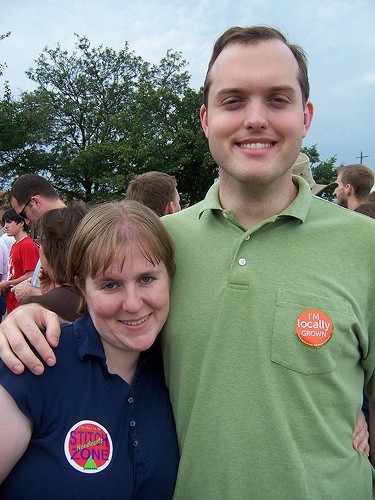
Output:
[33,238,46,248]
[19,197,32,219]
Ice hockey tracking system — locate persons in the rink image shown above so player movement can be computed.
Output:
[0,199,370,500]
[0,26,375,500]
[0,153,374,326]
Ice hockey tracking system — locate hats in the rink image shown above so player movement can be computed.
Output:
[291,153,339,195]
